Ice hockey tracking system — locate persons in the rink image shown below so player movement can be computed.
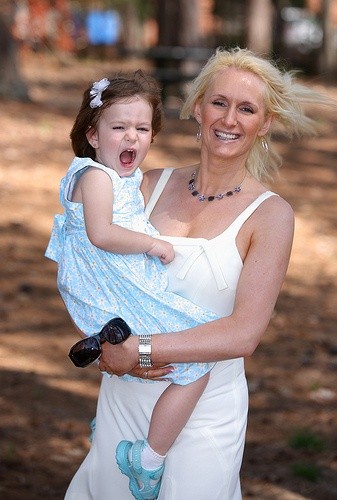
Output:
[45,77,214,500]
[59,52,296,499]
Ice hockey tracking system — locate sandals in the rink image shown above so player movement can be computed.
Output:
[87,415,95,444]
[115,438,165,500]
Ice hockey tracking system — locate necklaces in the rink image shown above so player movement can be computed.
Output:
[188,161,247,202]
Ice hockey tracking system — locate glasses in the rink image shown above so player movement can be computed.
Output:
[68,316,132,368]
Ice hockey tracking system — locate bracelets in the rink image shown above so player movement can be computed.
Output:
[138,335,150,370]
